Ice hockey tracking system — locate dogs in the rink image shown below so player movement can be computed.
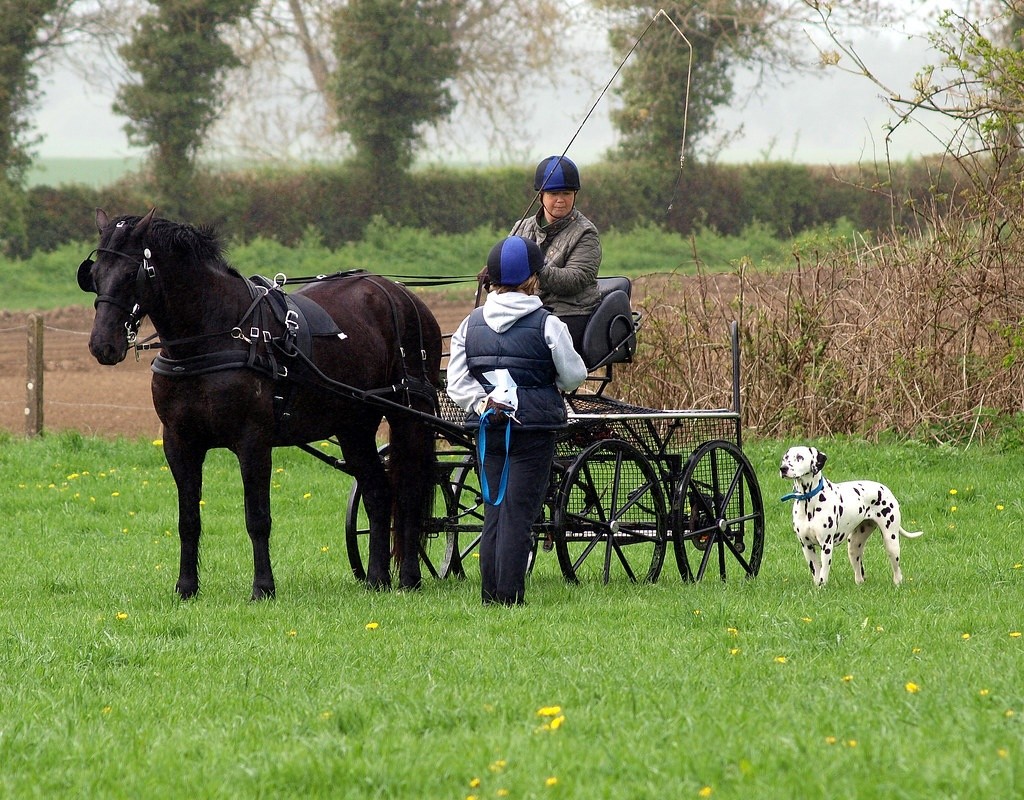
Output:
[779,446,924,590]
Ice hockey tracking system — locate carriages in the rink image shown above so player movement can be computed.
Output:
[76,205,767,602]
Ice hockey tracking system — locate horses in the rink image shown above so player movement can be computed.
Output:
[75,202,450,604]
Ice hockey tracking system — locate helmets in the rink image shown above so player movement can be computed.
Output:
[486,235,544,286]
[534,156,581,191]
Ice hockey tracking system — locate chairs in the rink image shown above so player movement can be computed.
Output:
[540,276,643,370]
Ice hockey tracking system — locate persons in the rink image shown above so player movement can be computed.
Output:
[447,236,588,608]
[477,156,602,351]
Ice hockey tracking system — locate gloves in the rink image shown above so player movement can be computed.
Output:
[484,399,516,430]
